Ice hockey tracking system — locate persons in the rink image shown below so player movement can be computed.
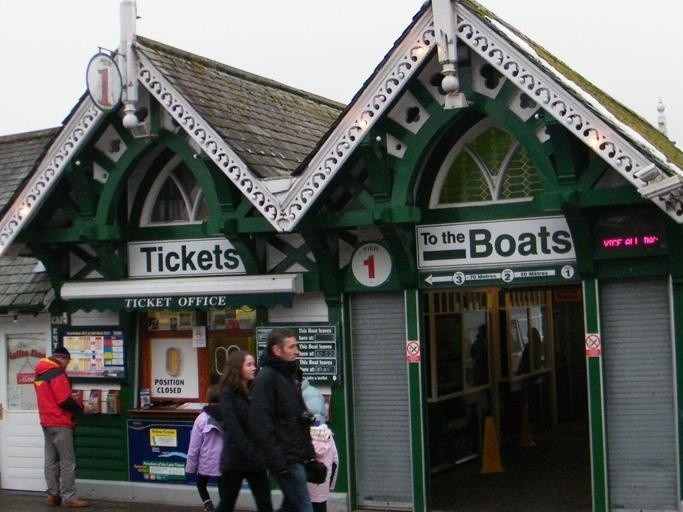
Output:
[246,327,318,510]
[185,384,225,510]
[515,326,548,374]
[32,346,90,507]
[214,350,273,511]
[470,323,488,384]
[298,379,338,511]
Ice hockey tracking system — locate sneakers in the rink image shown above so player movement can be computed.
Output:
[47,494,61,507]
[63,497,89,508]
[203,500,214,512]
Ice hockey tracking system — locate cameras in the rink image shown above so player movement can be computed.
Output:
[301,410,317,425]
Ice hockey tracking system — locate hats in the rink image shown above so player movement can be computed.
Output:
[52,347,70,359]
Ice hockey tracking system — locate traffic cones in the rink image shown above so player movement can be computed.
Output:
[478,414,508,477]
[513,394,539,449]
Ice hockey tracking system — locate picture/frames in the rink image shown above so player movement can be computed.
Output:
[139,329,210,405]
